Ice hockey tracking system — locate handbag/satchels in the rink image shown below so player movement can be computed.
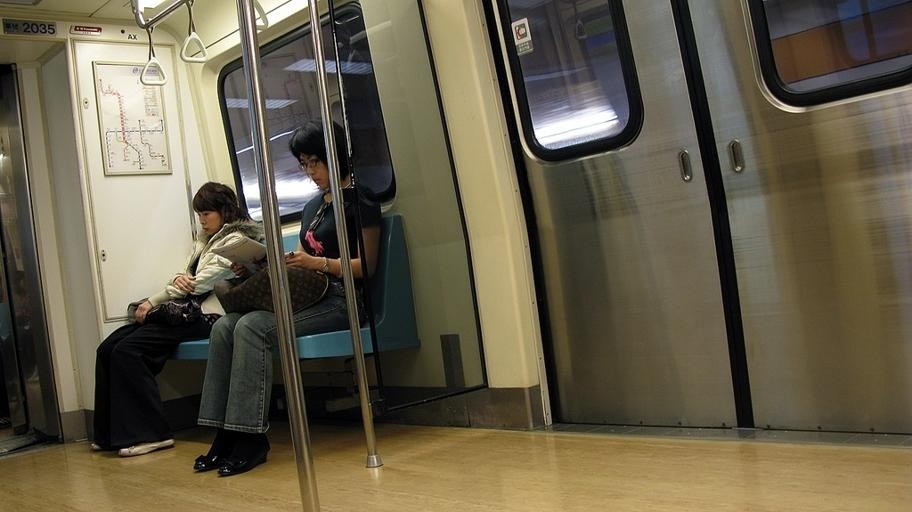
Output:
[214,265,328,316]
[127,297,204,325]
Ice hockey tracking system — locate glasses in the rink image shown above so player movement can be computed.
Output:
[297,158,320,171]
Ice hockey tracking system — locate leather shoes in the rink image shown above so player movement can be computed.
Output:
[91,443,102,450]
[117,439,175,457]
[194,441,272,475]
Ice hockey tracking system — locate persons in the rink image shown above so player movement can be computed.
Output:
[87,175,266,457]
[0,270,40,414]
[190,113,390,480]
[0,364,11,429]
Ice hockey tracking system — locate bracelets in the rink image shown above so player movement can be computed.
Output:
[320,256,330,274]
[147,299,154,309]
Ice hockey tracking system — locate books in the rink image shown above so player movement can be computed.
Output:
[209,230,267,270]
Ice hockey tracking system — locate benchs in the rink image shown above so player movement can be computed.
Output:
[177,214,421,412]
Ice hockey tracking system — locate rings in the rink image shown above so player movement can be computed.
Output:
[289,250,294,256]
